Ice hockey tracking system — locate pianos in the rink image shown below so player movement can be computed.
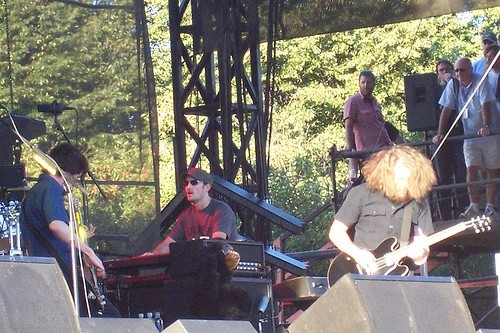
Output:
[270,275,329,302]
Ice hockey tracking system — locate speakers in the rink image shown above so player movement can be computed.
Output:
[231,278,275,333]
[404,73,445,131]
[79,317,160,333]
[160,319,258,333]
[285,272,476,333]
[0,255,86,333]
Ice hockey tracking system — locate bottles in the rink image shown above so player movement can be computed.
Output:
[138,311,164,333]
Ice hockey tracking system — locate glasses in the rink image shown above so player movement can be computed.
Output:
[453,67,472,72]
[183,179,199,187]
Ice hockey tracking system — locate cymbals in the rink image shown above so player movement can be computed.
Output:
[6,185,33,191]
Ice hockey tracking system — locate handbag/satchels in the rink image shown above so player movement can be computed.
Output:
[450,78,464,136]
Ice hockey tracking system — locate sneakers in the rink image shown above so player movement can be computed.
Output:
[484,205,498,215]
[458,205,483,219]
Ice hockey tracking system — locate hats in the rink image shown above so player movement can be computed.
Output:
[482,31,496,42]
[179,168,213,185]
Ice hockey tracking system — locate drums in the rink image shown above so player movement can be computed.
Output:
[0,204,22,240]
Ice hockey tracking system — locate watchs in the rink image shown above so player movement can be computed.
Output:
[483,124,491,128]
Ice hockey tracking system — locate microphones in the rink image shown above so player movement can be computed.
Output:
[38,101,75,113]
[257,296,269,316]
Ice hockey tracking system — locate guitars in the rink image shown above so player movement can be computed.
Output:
[326,212,499,289]
[69,190,103,306]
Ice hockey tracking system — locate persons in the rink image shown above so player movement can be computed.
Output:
[20,143,122,318]
[343,70,397,172]
[435,60,470,220]
[349,121,405,184]
[329,146,434,276]
[132,168,239,259]
[472,31,500,105]
[432,57,500,217]
